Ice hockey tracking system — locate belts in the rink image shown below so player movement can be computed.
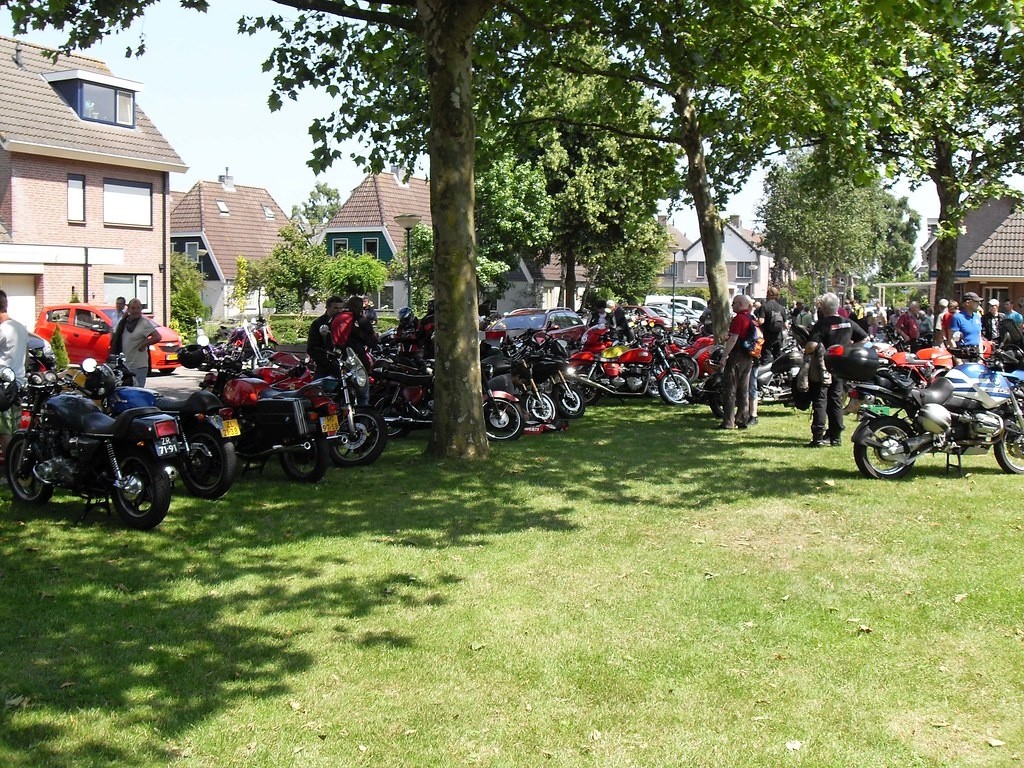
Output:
[964,345,978,348]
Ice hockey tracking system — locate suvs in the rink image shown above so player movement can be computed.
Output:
[487,307,586,341]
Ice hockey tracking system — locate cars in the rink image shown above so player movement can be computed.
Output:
[35,304,183,374]
[621,305,678,333]
[647,302,702,326]
[864,304,888,316]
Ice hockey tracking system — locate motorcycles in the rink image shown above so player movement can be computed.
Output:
[0,313,1024,529]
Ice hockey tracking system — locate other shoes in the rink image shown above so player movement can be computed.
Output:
[830,439,842,446]
[734,422,747,429]
[745,414,758,425]
[715,423,734,429]
[809,440,824,447]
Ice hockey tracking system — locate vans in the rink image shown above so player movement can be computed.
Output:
[645,294,709,312]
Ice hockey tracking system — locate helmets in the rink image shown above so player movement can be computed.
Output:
[619,327,634,342]
[86,362,115,400]
[398,308,414,328]
[918,403,952,433]
[27,336,46,361]
[176,344,203,369]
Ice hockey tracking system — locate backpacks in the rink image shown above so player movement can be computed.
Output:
[330,311,365,350]
[770,304,783,333]
[736,312,765,360]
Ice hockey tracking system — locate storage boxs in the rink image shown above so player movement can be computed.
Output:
[823,343,880,382]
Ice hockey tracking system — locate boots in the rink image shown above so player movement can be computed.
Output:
[0,434,13,464]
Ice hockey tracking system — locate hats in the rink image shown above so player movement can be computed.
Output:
[348,297,363,312]
[987,299,1000,307]
[938,298,949,307]
[962,292,984,302]
[426,300,435,318]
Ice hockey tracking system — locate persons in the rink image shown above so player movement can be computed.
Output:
[786,292,1024,371]
[398,305,434,375]
[700,300,713,335]
[758,285,785,365]
[333,296,380,406]
[718,294,766,430]
[478,299,493,317]
[0,290,29,466]
[121,298,163,387]
[111,310,128,356]
[587,300,634,348]
[307,297,344,380]
[804,292,869,447]
[111,297,128,338]
[361,296,377,326]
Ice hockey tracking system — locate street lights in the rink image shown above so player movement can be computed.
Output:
[748,265,758,299]
[850,274,861,300]
[667,245,683,334]
[393,213,422,307]
